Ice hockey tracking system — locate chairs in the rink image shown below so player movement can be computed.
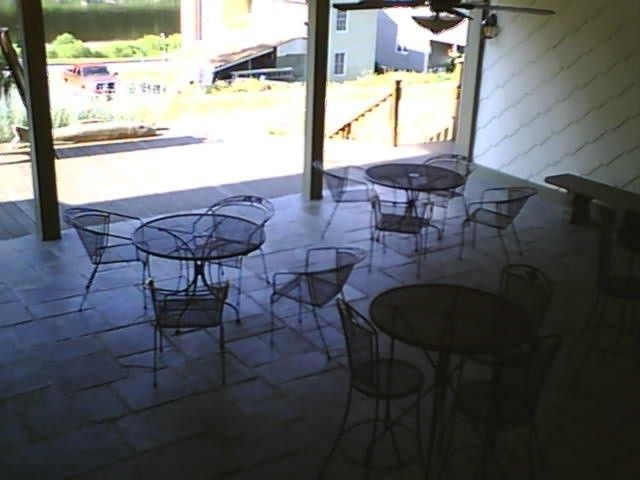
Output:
[311,159,384,242]
[143,276,234,393]
[417,154,477,236]
[314,264,567,478]
[577,211,637,364]
[269,245,372,363]
[366,194,436,280]
[63,205,155,313]
[189,193,276,325]
[455,186,539,267]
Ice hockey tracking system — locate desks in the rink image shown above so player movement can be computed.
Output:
[127,211,267,335]
[361,162,466,245]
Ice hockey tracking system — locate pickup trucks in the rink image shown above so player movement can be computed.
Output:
[62,61,119,99]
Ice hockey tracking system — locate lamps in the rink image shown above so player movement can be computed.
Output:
[412,13,466,34]
[481,15,500,39]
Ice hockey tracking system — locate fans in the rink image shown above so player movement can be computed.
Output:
[332,0,557,22]
